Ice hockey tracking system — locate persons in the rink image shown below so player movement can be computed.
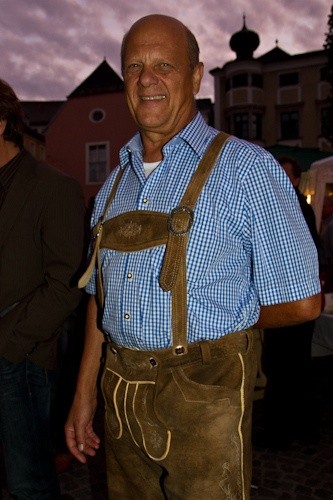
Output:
[0,78,102,500]
[62,14,333,500]
